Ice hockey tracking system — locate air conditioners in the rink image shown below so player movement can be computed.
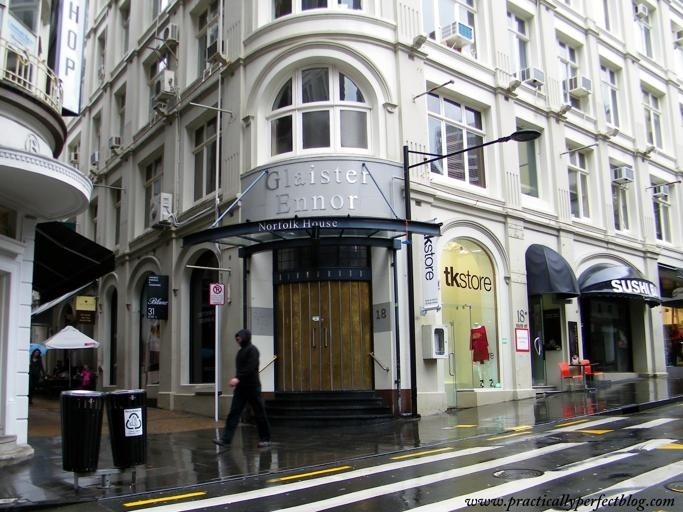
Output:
[522,65,545,88]
[70,151,80,162]
[97,63,105,80]
[108,135,121,149]
[570,75,593,98]
[441,20,475,50]
[675,28,683,46]
[159,22,178,51]
[612,166,635,184]
[652,184,670,200]
[90,151,99,164]
[634,3,649,18]
[148,192,173,230]
[150,68,175,100]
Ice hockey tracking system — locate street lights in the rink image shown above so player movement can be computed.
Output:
[402,128,542,418]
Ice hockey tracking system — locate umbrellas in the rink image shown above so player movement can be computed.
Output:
[30,342,47,354]
[45,326,100,387]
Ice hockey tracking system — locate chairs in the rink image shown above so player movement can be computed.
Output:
[580,360,606,391]
[557,362,586,393]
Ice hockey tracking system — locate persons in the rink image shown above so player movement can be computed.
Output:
[30,349,45,404]
[470,323,493,387]
[211,329,272,448]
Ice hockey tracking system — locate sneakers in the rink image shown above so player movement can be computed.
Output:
[211,440,231,448]
[257,441,271,447]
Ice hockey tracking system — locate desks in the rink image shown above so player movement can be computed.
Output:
[569,362,599,391]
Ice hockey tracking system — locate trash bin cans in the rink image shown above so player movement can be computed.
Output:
[105,389,148,467]
[60,390,104,474]
